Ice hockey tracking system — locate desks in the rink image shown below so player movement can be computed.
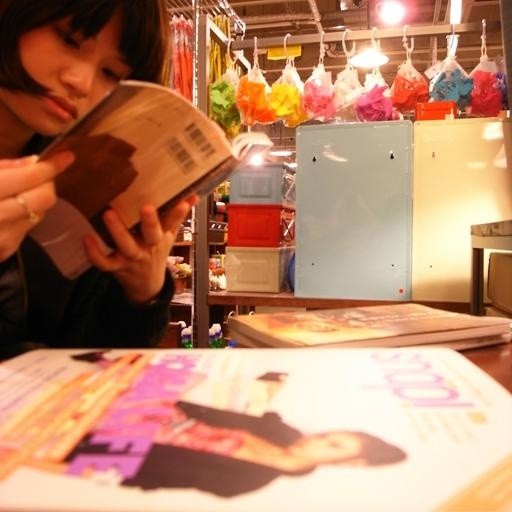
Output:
[470,218,512,318]
[209,285,468,321]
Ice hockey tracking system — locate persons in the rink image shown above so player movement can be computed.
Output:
[64,398,409,503]
[0,1,201,346]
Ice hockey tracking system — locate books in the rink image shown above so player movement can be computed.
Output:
[226,328,512,352]
[228,302,512,348]
[0,344,512,512]
[22,75,274,282]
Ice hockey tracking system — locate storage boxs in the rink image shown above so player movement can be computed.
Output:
[228,163,295,203]
[485,252,511,316]
[483,306,508,318]
[227,202,296,247]
[224,246,296,294]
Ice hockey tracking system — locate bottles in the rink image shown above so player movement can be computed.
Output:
[179,321,224,348]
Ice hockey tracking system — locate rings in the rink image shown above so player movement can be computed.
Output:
[15,194,40,222]
